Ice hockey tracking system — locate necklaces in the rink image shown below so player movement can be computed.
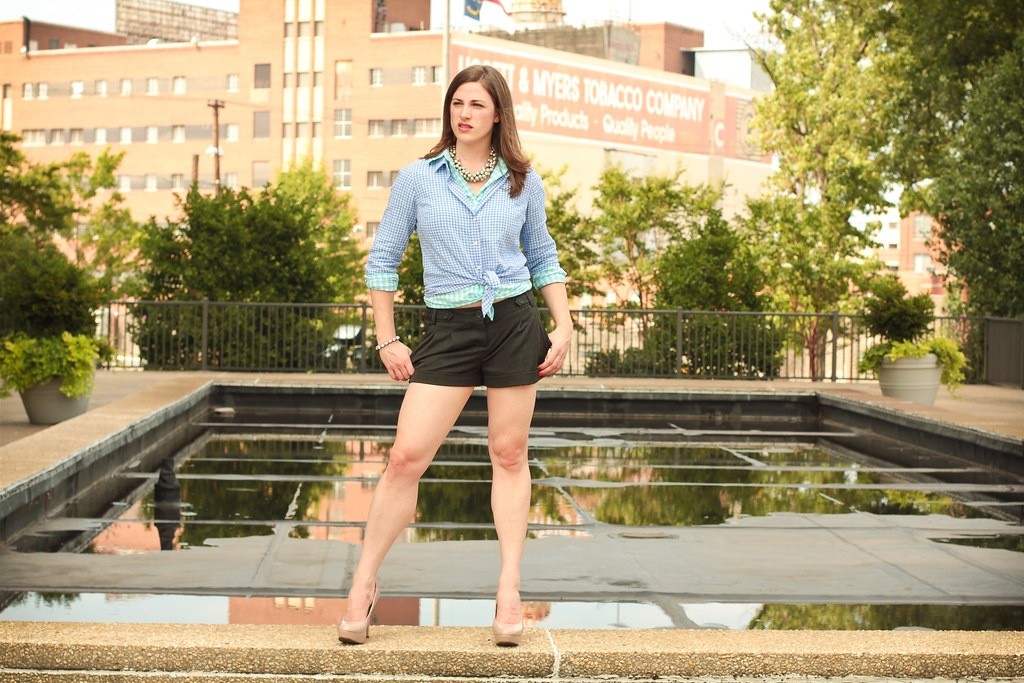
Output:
[448,143,499,182]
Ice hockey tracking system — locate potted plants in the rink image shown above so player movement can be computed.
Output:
[0,329,118,425]
[857,336,974,408]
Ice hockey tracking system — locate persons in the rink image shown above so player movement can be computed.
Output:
[336,63,578,648]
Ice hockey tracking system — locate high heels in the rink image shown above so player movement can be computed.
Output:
[338,577,380,644]
[493,594,523,646]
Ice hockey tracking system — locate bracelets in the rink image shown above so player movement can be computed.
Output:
[375,335,400,351]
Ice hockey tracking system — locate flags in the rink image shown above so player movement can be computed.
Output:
[463,0,519,38]
